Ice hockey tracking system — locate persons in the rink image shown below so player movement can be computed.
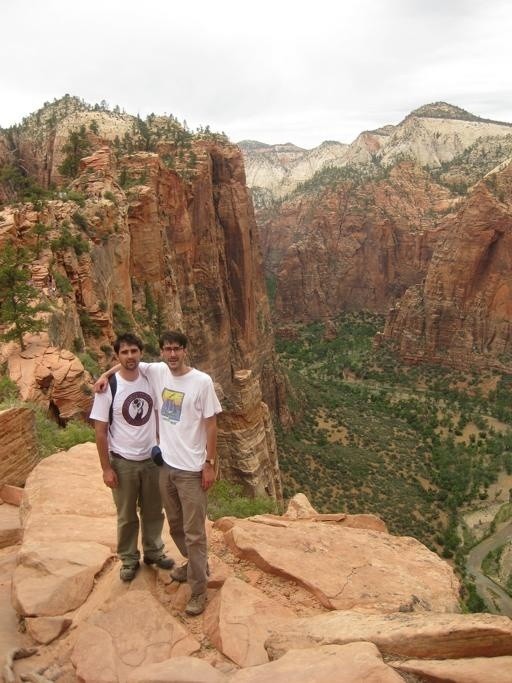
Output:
[88,334,174,581]
[94,330,222,616]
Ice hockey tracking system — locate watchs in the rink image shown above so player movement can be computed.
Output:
[206,459,216,466]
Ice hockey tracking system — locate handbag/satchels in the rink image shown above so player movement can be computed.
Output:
[151,446,163,466]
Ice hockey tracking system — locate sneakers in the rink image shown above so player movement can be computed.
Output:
[184,592,207,616]
[143,554,175,569]
[120,560,139,580]
[170,563,210,582]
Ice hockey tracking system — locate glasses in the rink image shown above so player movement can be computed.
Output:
[162,347,186,353]
